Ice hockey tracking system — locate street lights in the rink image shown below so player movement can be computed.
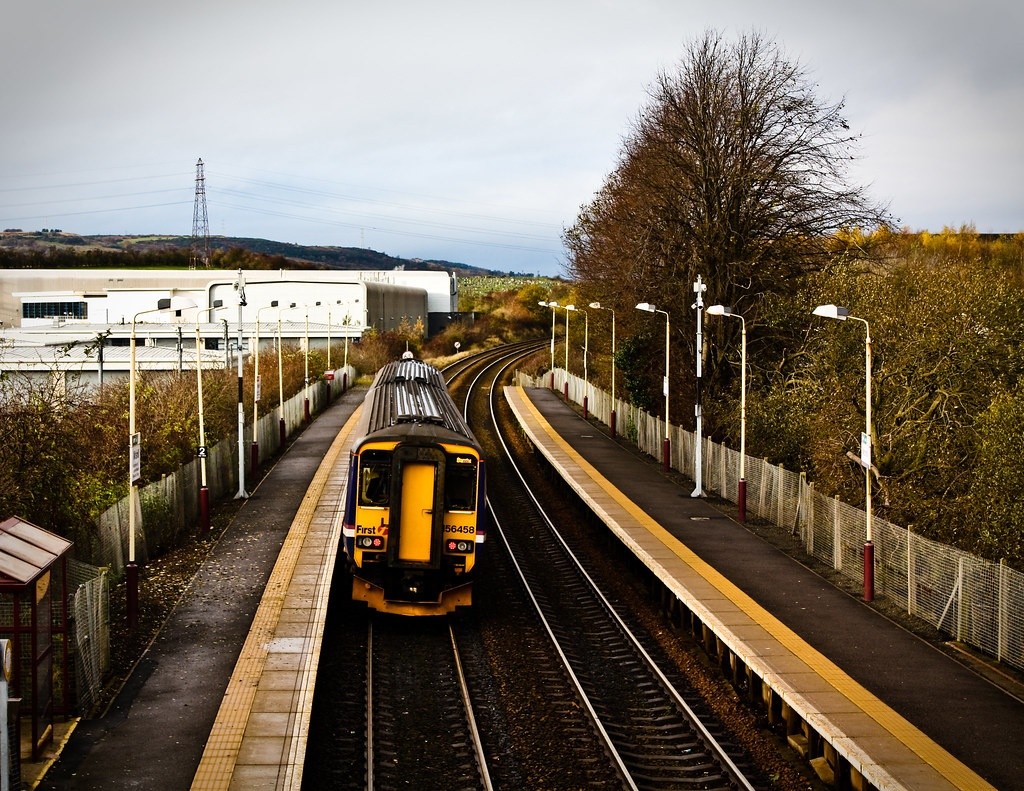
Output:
[126,294,198,627]
[326,299,348,405]
[342,297,363,393]
[275,301,309,452]
[811,302,875,603]
[193,297,240,534]
[537,300,557,392]
[549,301,570,402]
[564,304,589,419]
[705,304,748,527]
[303,298,333,425]
[249,298,291,479]
[633,301,672,473]
[588,301,617,438]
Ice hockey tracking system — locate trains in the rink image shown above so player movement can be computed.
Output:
[338,356,490,619]
